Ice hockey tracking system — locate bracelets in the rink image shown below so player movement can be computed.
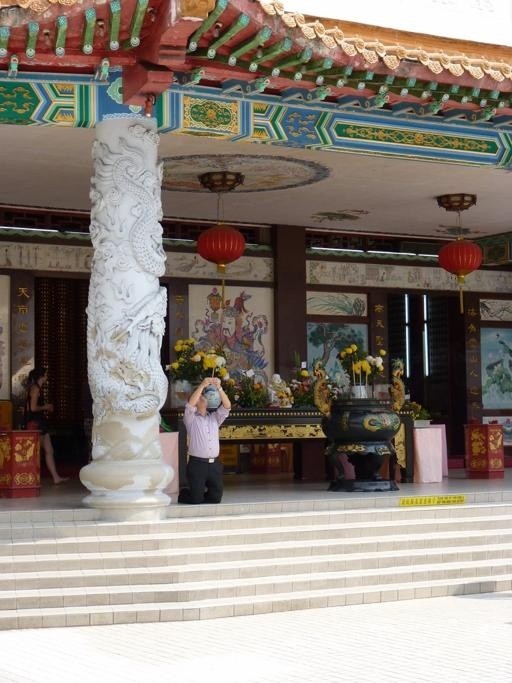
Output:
[219,386,222,392]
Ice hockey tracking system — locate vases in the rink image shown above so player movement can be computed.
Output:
[349,385,368,398]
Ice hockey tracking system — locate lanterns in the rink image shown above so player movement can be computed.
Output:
[438,237,482,313]
[198,222,245,309]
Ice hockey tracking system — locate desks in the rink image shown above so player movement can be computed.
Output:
[159,409,414,487]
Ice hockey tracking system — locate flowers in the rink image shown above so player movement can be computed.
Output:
[165,338,271,407]
[336,343,386,385]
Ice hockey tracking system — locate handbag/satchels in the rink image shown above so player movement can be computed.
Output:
[20,410,50,435]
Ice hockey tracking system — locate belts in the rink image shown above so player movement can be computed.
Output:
[190,455,219,464]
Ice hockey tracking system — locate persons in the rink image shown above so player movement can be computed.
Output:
[199,291,255,372]
[24,368,71,485]
[268,374,293,405]
[179,377,232,504]
